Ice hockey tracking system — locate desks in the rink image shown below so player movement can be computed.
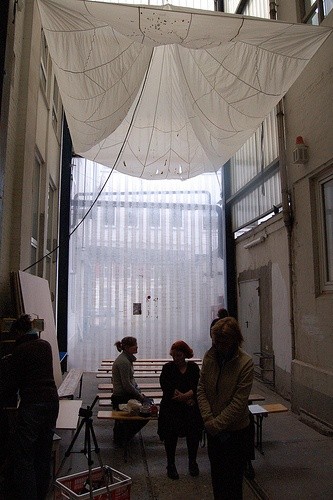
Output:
[56,400,82,469]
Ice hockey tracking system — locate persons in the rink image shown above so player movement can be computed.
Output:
[0,314,60,499]
[157,341,204,479]
[210,309,228,339]
[110,337,153,444]
[196,316,254,500]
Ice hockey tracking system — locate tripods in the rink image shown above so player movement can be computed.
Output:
[45,396,115,500]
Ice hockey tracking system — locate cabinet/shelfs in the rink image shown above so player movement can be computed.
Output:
[253,352,275,388]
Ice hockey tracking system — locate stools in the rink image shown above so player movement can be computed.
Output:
[52,434,61,491]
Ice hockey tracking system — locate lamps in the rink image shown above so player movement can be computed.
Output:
[243,236,266,250]
[292,135,308,164]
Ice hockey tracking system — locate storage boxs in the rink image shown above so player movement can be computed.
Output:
[56,465,133,500]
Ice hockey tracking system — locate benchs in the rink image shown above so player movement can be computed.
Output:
[96,358,289,464]
[58,367,84,400]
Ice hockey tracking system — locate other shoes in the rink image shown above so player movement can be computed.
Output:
[188,461,199,476]
[166,465,179,479]
